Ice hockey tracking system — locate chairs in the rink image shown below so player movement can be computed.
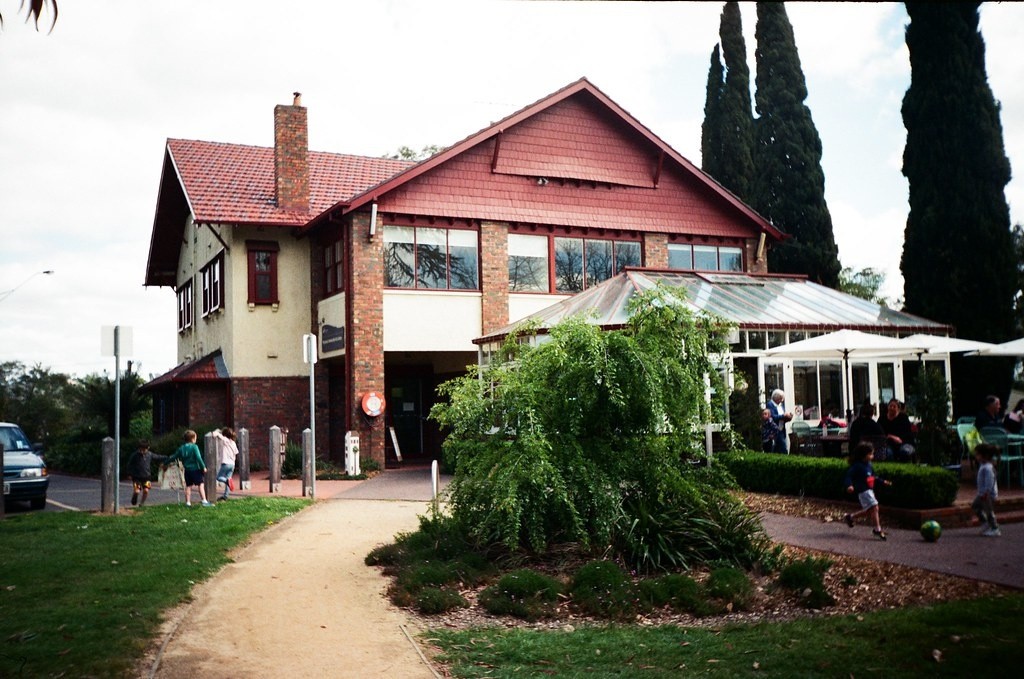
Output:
[789,423,812,454]
[957,419,1024,493]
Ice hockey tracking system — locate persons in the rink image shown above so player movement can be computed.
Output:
[215,426,240,502]
[735,380,748,394]
[877,398,915,462]
[1002,398,1024,434]
[169,430,215,507]
[817,416,841,428]
[760,388,793,454]
[849,398,902,463]
[125,439,170,508]
[761,408,779,454]
[844,440,892,542]
[974,395,1000,433]
[972,443,1001,537]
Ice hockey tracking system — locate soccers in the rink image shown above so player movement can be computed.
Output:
[919,521,944,542]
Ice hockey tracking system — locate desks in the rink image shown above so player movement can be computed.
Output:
[815,435,850,458]
[986,434,1024,492]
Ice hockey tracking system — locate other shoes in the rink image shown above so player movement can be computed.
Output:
[873,529,886,540]
[202,500,214,507]
[186,504,191,508]
[984,528,1001,536]
[132,496,137,505]
[846,513,855,527]
[227,477,234,490]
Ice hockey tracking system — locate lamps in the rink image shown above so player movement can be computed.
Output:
[538,177,549,186]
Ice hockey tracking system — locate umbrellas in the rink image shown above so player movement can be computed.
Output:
[762,328,1024,422]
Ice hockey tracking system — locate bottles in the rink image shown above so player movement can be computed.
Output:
[823,424,827,436]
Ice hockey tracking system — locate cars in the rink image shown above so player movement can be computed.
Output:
[0,422,51,512]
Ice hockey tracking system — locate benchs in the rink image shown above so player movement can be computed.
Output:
[810,426,847,449]
